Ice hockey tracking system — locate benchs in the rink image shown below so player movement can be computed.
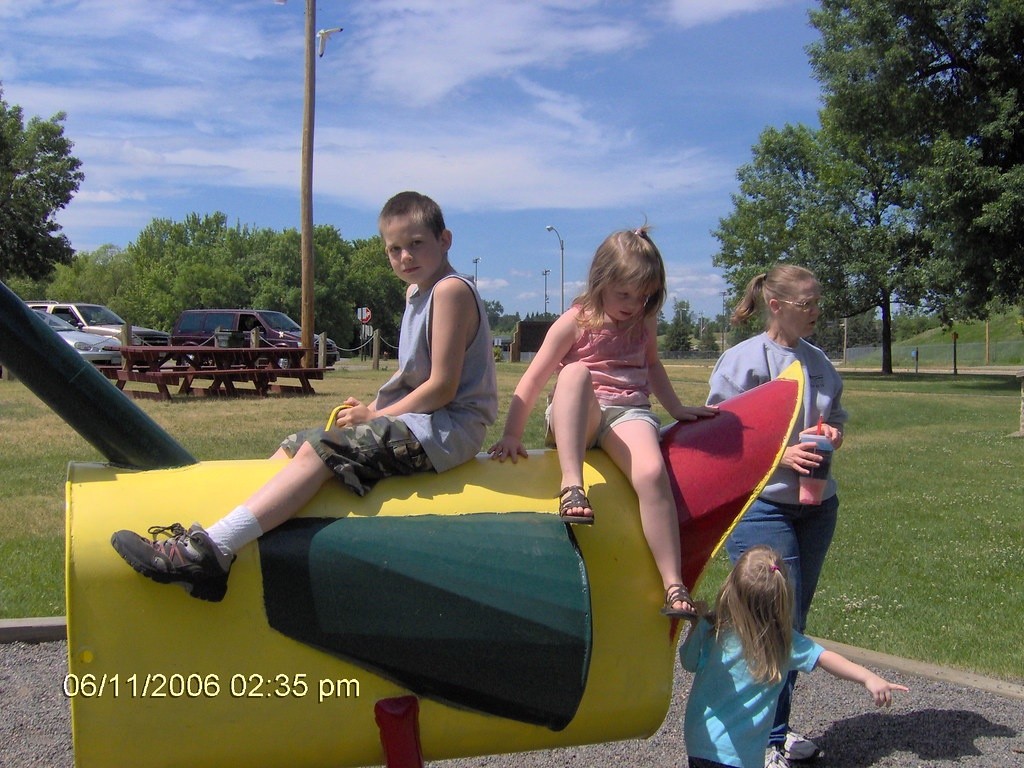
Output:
[145,365,335,400]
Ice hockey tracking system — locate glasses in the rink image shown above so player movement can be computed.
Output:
[778,295,825,310]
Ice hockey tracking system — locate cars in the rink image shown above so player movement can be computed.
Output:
[31,308,123,369]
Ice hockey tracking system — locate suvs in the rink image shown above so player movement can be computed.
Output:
[173,309,339,375]
[26,300,173,371]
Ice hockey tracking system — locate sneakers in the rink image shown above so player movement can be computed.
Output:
[111,522,238,603]
[764,731,820,768]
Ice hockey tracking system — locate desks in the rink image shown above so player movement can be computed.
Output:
[102,345,318,401]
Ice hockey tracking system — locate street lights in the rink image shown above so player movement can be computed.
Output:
[547,226,565,315]
[473,257,482,287]
[542,270,551,314]
[719,291,726,352]
[699,311,705,340]
[679,308,688,324]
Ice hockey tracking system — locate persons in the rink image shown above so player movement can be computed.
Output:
[705,265,852,768]
[679,544,908,768]
[487,212,720,619]
[110,193,498,601]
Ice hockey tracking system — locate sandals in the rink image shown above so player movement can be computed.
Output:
[552,485,595,525]
[660,583,700,620]
